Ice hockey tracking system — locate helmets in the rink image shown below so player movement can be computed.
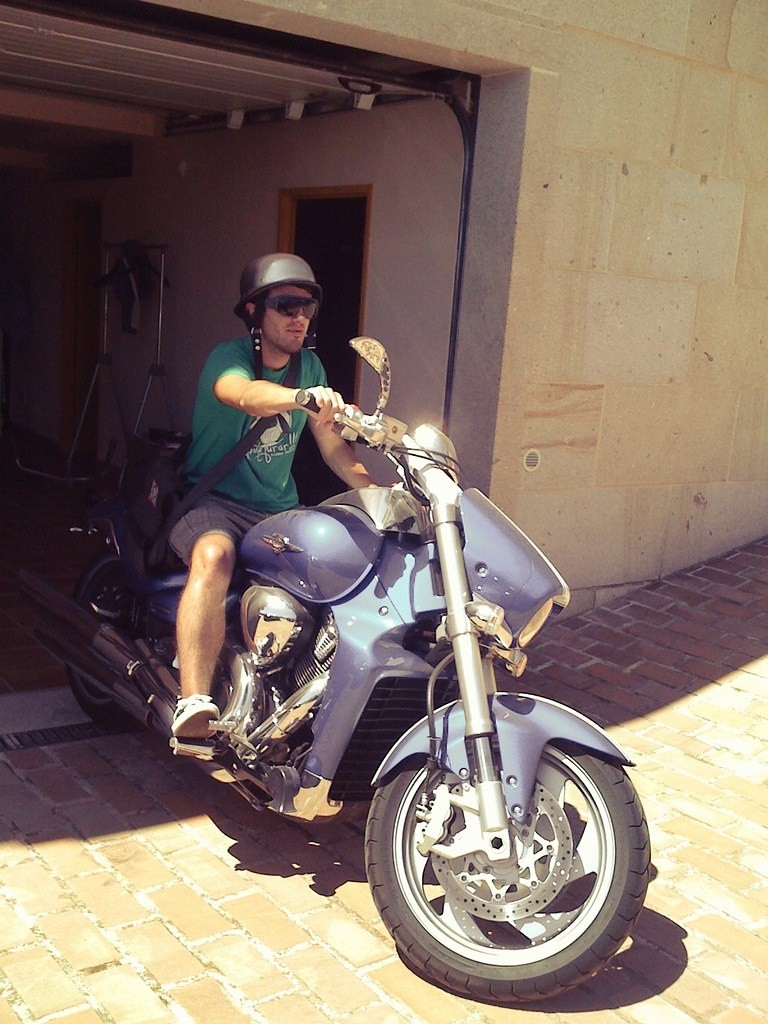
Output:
[234,252,323,318]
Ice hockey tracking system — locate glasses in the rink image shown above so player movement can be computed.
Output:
[265,295,319,319]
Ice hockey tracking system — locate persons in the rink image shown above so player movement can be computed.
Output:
[164,252,376,738]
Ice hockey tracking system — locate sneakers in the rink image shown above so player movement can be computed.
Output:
[171,694,221,739]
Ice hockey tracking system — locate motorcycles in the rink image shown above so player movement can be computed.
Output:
[28,336,651,1009]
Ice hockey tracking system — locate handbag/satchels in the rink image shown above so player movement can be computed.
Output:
[125,458,186,572]
